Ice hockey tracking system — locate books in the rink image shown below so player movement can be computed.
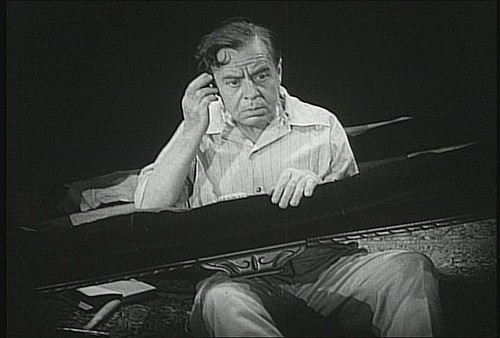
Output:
[57,278,158,313]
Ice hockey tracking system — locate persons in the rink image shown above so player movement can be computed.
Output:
[133,20,443,338]
